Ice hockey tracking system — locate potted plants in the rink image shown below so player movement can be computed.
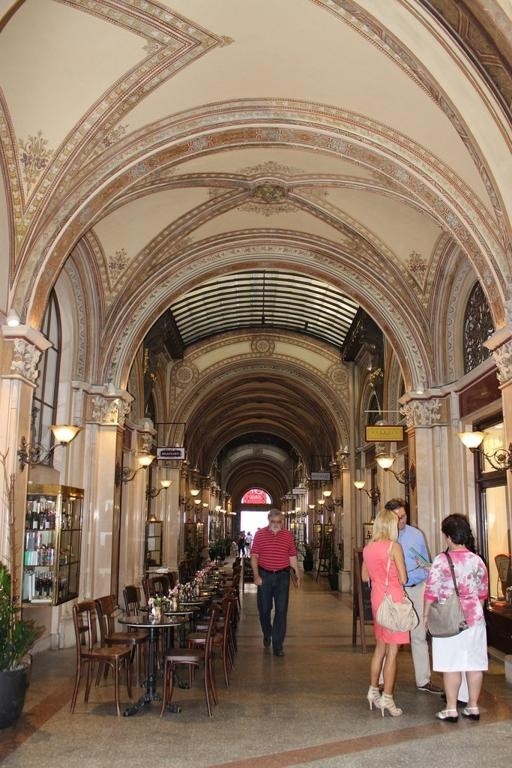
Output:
[1,558,47,730]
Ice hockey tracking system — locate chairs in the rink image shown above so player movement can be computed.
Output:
[67,556,245,718]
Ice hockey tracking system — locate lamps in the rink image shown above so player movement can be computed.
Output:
[296,489,344,518]
[455,428,512,475]
[18,422,83,472]
[178,486,209,515]
[374,449,418,492]
[352,480,382,503]
[145,480,175,501]
[115,452,158,490]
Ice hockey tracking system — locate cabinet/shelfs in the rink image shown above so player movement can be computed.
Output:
[21,484,85,607]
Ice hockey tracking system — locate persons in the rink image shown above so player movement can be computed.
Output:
[227,527,261,557]
[418,531,479,706]
[251,509,302,657]
[361,510,414,716]
[376,499,445,698]
[424,514,490,722]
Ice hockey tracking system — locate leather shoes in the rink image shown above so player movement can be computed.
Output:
[418,682,443,694]
[263,626,272,647]
[273,645,284,656]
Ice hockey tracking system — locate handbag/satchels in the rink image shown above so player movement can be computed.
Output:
[376,594,418,631]
[428,592,468,636]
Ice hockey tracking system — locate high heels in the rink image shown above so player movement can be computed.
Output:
[367,686,403,717]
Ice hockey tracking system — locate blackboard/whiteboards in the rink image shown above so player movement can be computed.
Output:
[354,547,373,626]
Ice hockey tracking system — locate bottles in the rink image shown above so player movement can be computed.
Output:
[27,499,76,601]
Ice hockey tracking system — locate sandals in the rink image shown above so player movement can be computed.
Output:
[435,709,458,722]
[461,707,479,721]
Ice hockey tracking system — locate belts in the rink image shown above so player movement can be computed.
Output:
[259,567,287,573]
